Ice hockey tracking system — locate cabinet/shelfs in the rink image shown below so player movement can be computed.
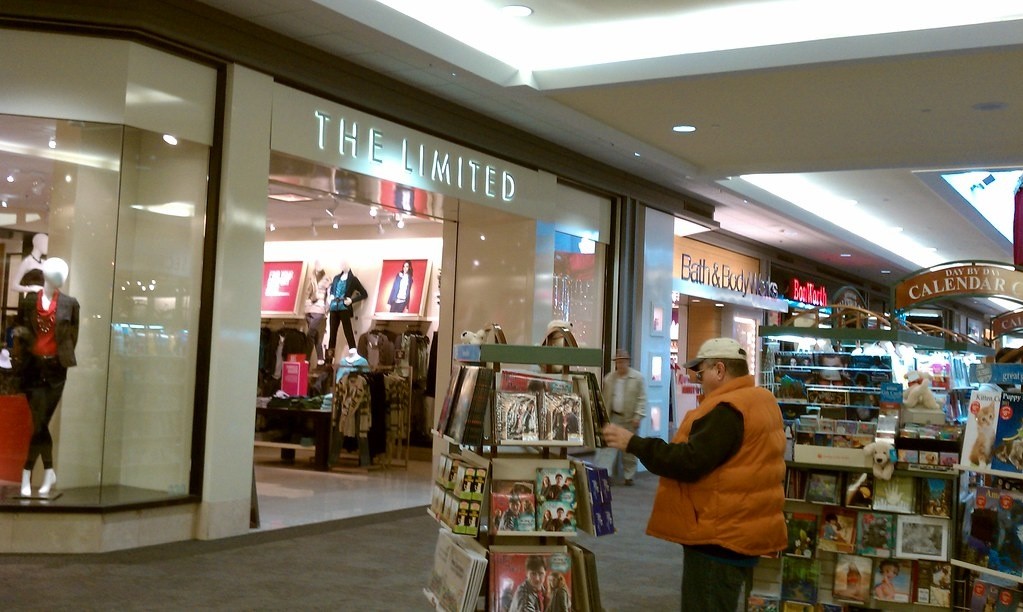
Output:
[430,344,614,612]
[747,299,1021,611]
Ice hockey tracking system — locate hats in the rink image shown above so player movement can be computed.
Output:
[682,338,747,372]
[611,349,633,361]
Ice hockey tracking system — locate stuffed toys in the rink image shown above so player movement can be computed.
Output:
[460,324,492,368]
[866,441,898,480]
[902,372,940,411]
[535,321,576,374]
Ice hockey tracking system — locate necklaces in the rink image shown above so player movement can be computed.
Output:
[28,252,43,263]
[38,289,57,333]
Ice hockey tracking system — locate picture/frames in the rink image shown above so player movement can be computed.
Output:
[368,254,434,319]
[262,258,308,316]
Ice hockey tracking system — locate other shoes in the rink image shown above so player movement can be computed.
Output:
[625,479,634,485]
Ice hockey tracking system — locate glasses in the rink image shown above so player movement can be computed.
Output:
[695,364,717,381]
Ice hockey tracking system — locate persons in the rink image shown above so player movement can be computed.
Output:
[304,258,331,367]
[336,344,371,394]
[496,380,577,612]
[10,257,80,496]
[387,260,414,314]
[12,233,49,327]
[326,258,368,361]
[602,337,788,612]
[601,348,648,487]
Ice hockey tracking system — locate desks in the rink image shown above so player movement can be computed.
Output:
[256,396,339,471]
